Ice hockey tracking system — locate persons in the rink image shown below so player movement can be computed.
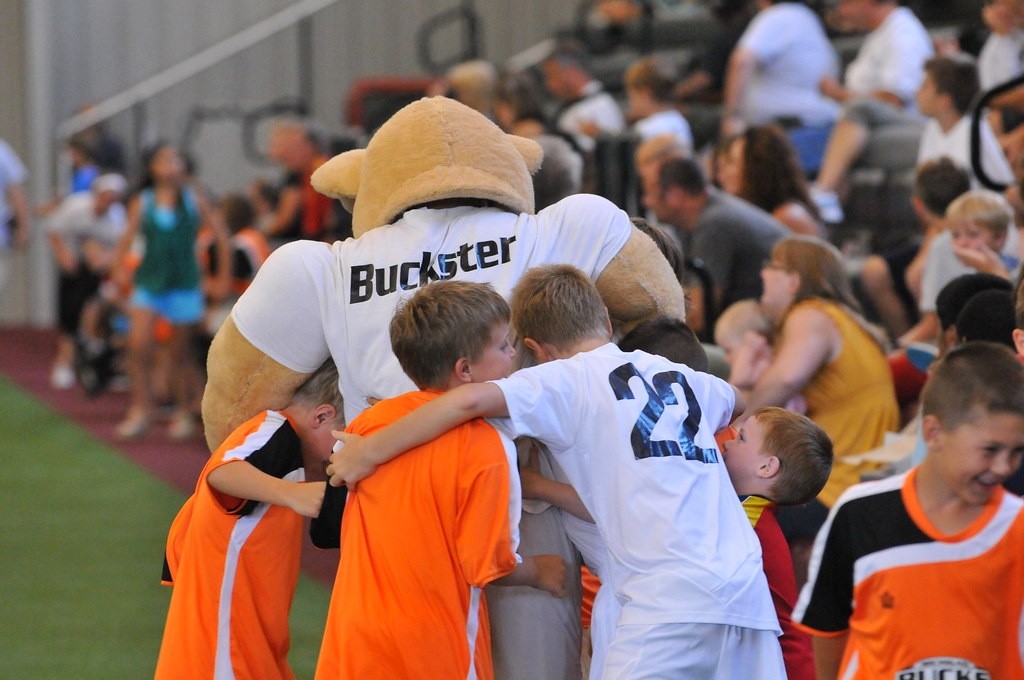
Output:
[53,0,1024,558]
[314,280,568,680]
[722,406,834,680]
[793,345,1024,680]
[0,138,33,254]
[154,356,347,680]
[324,263,788,680]
[200,94,689,680]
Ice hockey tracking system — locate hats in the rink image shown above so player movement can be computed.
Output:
[92,173,128,201]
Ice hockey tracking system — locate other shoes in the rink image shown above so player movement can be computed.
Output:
[113,412,157,437]
[165,412,197,440]
[810,191,843,223]
[51,359,76,387]
